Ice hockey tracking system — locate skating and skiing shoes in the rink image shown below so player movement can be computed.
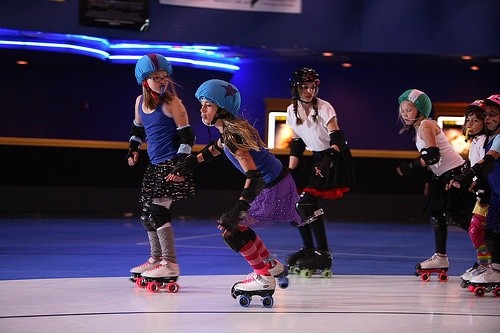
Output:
[129,257,168,289]
[140,260,179,293]
[469,263,500,297]
[231,271,276,308]
[415,253,449,283]
[296,251,333,279]
[286,248,317,275]
[460,265,491,288]
[246,258,290,289]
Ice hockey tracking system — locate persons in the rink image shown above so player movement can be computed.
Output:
[185,79,304,308]
[285,67,351,278]
[126,53,195,293]
[445,94,500,298]
[397,89,474,282]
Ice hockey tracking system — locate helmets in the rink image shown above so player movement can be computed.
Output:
[486,94,500,105]
[194,79,241,116]
[135,54,172,85]
[289,67,320,82]
[398,89,432,117]
[465,100,487,116]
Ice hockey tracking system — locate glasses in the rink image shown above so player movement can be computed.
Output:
[299,85,317,89]
[148,76,169,82]
